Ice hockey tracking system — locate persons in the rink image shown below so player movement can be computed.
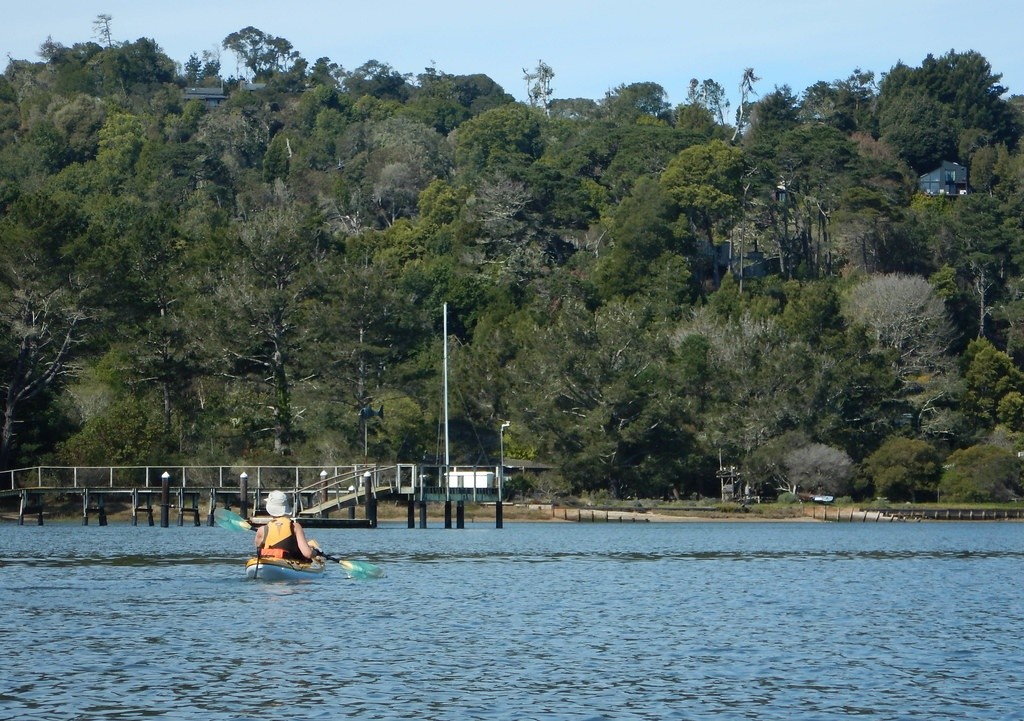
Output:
[255,490,324,565]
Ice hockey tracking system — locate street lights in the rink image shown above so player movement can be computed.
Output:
[500,421,511,489]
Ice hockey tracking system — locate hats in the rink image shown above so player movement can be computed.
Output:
[263,490,293,517]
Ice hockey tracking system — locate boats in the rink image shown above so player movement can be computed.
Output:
[244,556,325,580]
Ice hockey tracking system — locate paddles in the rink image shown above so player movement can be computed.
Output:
[213,507,384,580]
